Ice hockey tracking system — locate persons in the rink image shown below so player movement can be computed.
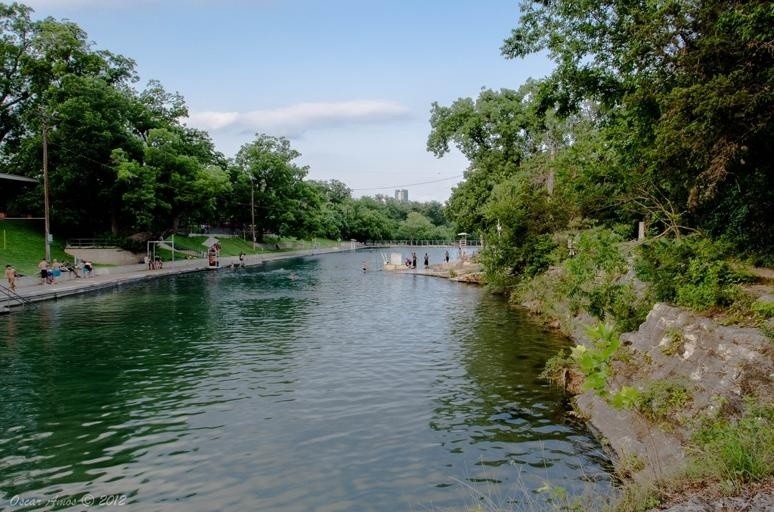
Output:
[444,250,449,263]
[410,252,416,269]
[456,247,477,264]
[38,257,92,287]
[3,264,20,296]
[239,251,242,265]
[212,244,217,252]
[241,253,246,266]
[209,257,219,266]
[423,252,429,269]
[405,258,411,265]
[142,255,163,270]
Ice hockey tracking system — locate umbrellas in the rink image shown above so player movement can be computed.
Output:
[457,232,471,237]
